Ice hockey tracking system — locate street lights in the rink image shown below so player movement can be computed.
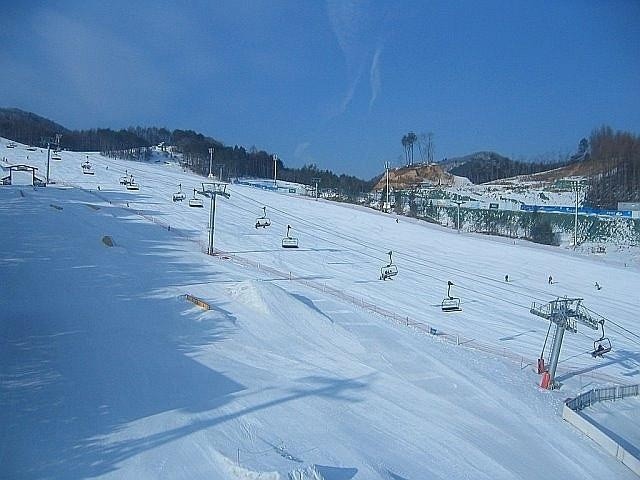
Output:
[383,160,391,203]
[272,152,279,187]
[209,146,214,179]
[570,179,584,247]
